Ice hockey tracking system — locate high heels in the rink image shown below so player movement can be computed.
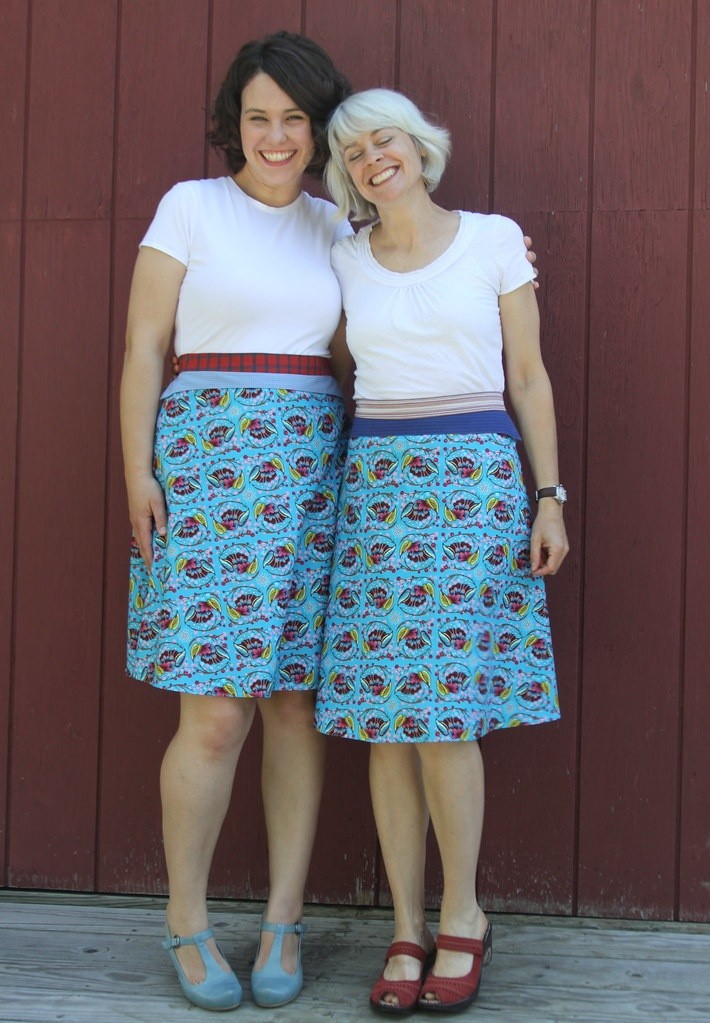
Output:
[249,905,306,1008]
[420,923,494,1012]
[160,920,243,1011]
[369,938,437,1018]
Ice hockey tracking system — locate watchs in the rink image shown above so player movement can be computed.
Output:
[533,483,568,506]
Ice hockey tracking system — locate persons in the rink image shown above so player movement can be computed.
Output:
[318,87,572,1016]
[119,32,539,1012]
